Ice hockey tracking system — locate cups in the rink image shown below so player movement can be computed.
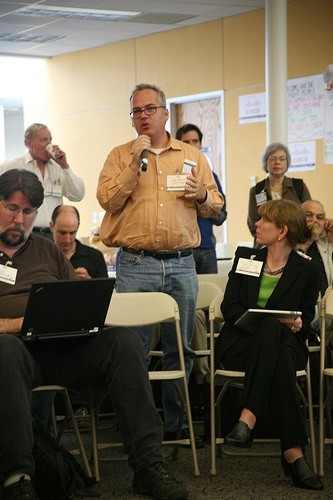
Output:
[46,144,60,160]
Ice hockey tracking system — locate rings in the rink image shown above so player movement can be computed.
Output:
[292,326,295,330]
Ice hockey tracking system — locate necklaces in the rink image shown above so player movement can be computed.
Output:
[265,262,285,275]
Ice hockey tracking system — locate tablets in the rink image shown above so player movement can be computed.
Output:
[235,309,302,327]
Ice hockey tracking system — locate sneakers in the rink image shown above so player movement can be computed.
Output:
[3,475,40,500]
[132,465,189,500]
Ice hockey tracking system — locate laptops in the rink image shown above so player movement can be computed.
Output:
[14,277,116,342]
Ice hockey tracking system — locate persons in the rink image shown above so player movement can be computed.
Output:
[44,204,109,278]
[207,197,329,489]
[97,83,225,448]
[247,144,312,248]
[0,123,86,242]
[296,199,333,369]
[176,124,228,334]
[0,169,189,498]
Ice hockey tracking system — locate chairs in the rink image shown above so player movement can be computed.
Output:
[28,264,333,481]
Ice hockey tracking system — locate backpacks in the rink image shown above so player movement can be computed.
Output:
[29,410,94,500]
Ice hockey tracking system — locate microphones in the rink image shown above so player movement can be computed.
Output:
[140,149,149,171]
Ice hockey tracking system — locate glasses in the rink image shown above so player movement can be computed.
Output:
[1,200,39,218]
[129,106,165,119]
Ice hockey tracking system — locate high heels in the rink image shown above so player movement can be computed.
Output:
[280,449,323,490]
[224,421,256,452]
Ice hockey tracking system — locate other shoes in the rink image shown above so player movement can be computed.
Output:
[167,432,204,448]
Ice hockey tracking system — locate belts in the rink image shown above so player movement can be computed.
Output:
[32,227,51,233]
[121,246,193,260]
